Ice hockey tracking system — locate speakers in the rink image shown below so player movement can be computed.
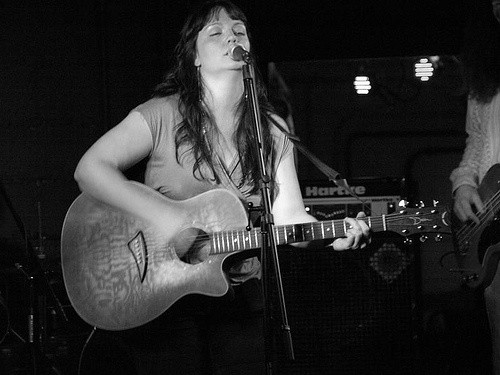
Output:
[263,234,426,375]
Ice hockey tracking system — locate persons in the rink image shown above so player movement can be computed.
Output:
[75,0,371,375]
[449,0,500,375]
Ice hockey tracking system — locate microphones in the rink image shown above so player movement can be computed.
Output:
[229,44,251,63]
[15,263,31,280]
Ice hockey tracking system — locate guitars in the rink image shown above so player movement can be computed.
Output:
[449,163,500,290]
[61,181,454,331]
